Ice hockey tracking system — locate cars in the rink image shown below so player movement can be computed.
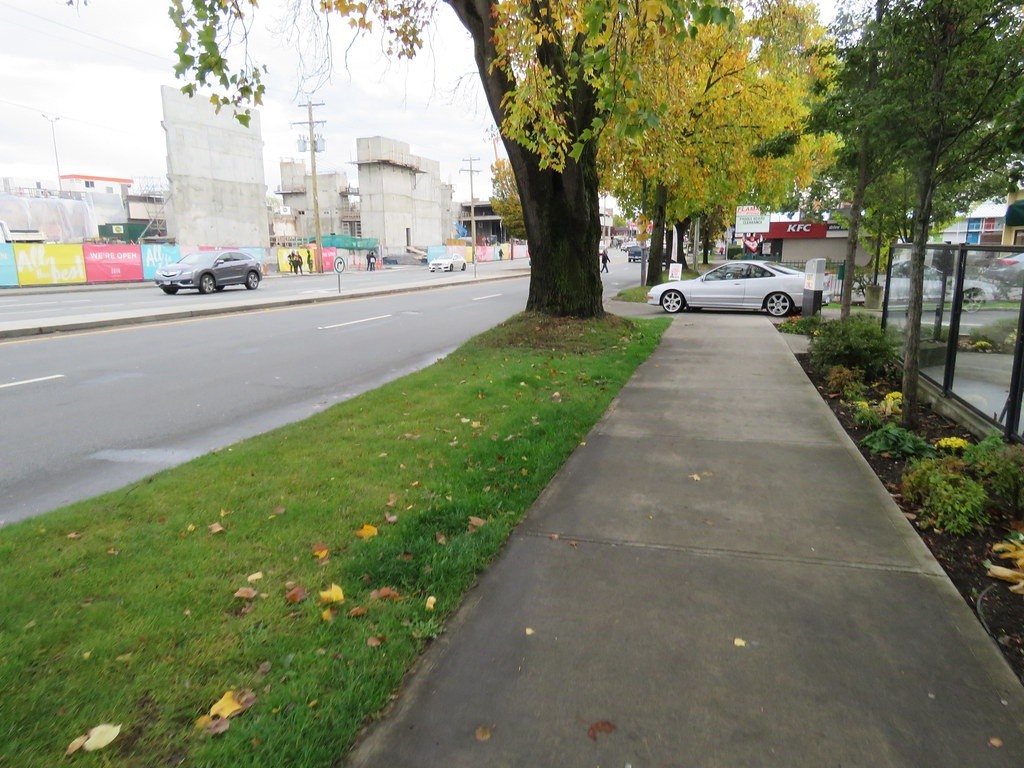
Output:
[154,249,264,295]
[647,259,833,317]
[429,253,466,272]
[628,245,643,261]
[621,242,626,251]
[983,252,1024,284]
[872,261,993,313]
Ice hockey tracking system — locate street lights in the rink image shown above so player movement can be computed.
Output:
[42,113,63,189]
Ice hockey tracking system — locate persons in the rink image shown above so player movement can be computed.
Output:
[367,251,376,271]
[720,246,724,255]
[599,250,610,273]
[288,250,303,275]
[307,250,313,273]
[498,249,504,260]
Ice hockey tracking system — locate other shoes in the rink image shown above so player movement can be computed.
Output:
[606,271,608,273]
[600,271,602,273]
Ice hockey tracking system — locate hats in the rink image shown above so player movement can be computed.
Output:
[824,272,830,275]
